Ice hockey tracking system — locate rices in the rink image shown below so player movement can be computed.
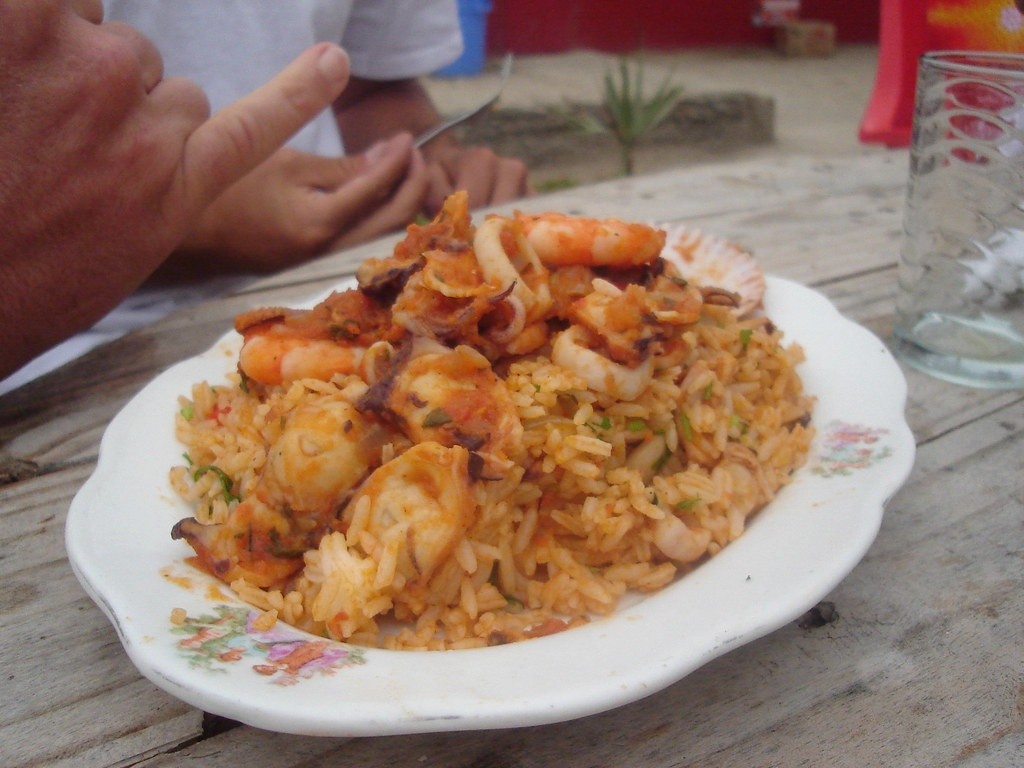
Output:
[173,268,818,669]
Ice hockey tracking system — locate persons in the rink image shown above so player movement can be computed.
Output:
[0,0,531,400]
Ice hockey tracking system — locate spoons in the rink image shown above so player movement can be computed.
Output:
[414,49,514,158]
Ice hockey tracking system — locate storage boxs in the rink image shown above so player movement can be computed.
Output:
[784,22,834,58]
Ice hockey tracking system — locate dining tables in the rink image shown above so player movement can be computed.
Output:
[0,138,1024,768]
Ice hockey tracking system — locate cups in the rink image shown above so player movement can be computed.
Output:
[890,50,1023,393]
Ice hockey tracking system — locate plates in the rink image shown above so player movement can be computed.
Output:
[66,258,920,736]
